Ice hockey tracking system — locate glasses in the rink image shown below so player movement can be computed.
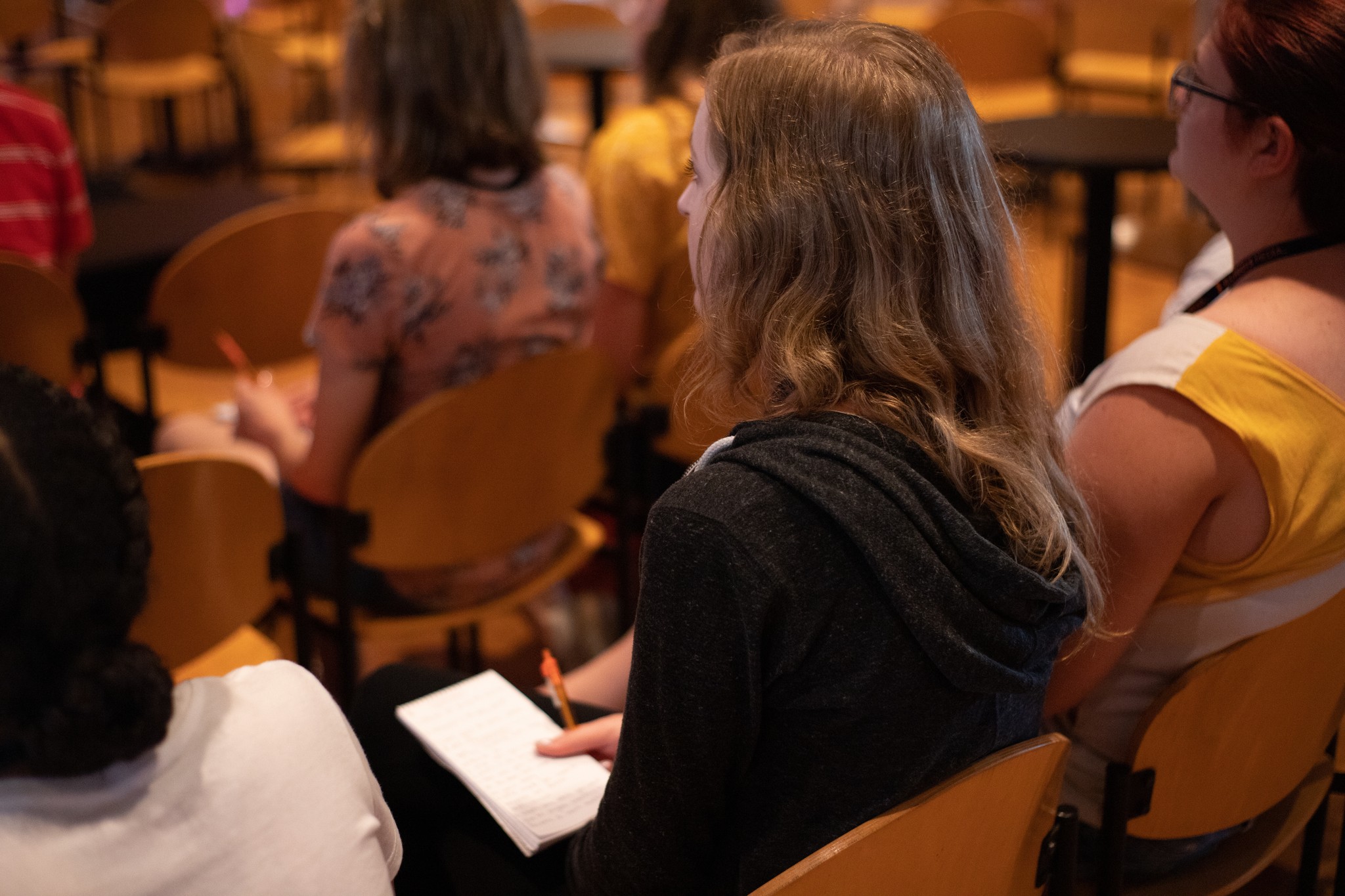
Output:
[1169,56,1258,120]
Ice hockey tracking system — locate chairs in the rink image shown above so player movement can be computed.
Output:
[0,0,1345,896]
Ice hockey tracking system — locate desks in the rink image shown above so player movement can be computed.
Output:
[64,182,291,442]
[529,24,647,136]
[980,111,1179,399]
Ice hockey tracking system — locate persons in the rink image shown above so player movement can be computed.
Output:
[1052,0,1345,896]
[339,19,1106,896]
[0,361,403,896]
[156,1,599,619]
[0,44,95,278]
[582,0,786,498]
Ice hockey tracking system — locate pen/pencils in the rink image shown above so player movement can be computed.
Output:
[214,331,269,380]
[541,648,580,730]
